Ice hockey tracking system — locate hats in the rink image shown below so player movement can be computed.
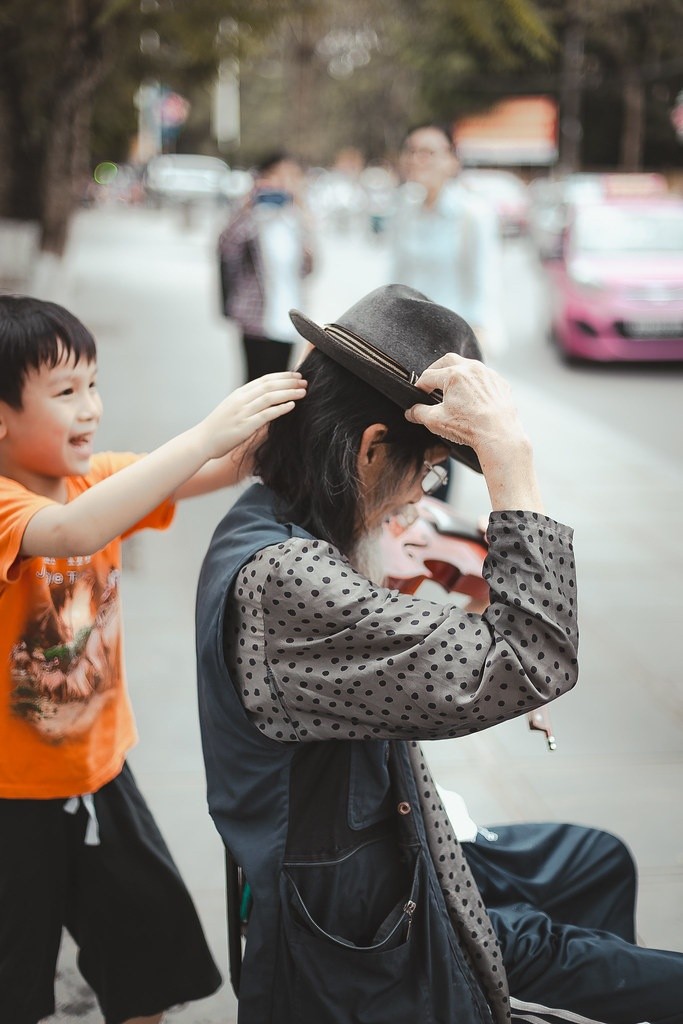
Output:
[288,285,485,474]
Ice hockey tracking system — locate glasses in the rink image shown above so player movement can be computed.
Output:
[420,462,449,496]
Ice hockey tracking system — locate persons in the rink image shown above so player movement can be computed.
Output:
[219,125,497,385]
[196,283,683,1023]
[0,293,308,1024]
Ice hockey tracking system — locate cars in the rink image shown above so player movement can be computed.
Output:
[463,168,683,367]
